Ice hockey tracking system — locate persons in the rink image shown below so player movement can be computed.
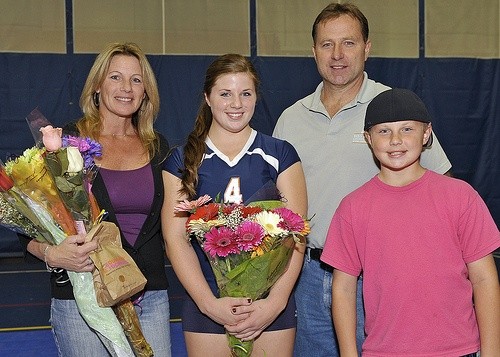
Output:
[272,4,454,357]
[161,53,310,357]
[18,41,176,357]
[319,87,500,357]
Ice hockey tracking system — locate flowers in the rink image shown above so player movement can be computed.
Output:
[172,193,316,356]
[1,122,154,356]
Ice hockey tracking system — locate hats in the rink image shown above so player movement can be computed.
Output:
[363,86,434,133]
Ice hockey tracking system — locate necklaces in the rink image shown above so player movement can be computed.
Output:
[102,127,138,137]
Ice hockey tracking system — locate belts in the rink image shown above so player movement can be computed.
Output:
[306,247,328,266]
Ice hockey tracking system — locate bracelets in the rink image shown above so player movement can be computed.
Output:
[45,245,58,272]
